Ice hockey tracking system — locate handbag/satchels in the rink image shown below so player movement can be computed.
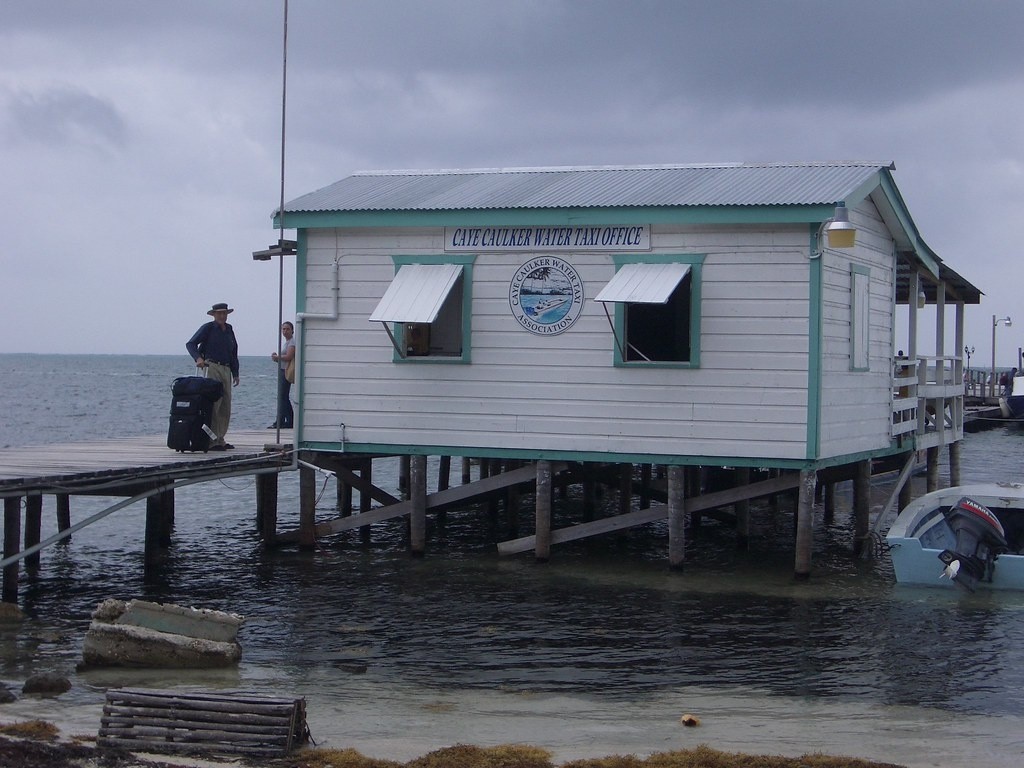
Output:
[199,322,212,359]
[170,376,224,402]
[284,358,295,383]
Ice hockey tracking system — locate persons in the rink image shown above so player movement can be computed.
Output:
[894,350,1017,396]
[267,322,296,428]
[185,303,240,451]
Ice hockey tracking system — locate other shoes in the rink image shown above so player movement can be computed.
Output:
[210,445,226,451]
[266,425,276,428]
[281,422,291,428]
[224,444,235,449]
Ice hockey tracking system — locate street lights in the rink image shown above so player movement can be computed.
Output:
[991,314,1012,397]
[965,344,975,381]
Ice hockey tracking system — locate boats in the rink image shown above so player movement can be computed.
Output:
[1006,347,1024,420]
[886,480,1024,592]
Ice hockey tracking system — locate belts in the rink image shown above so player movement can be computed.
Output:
[209,360,230,367]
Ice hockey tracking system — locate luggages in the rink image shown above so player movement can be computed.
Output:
[166,363,212,453]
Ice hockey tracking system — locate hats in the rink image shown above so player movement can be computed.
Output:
[207,304,233,316]
